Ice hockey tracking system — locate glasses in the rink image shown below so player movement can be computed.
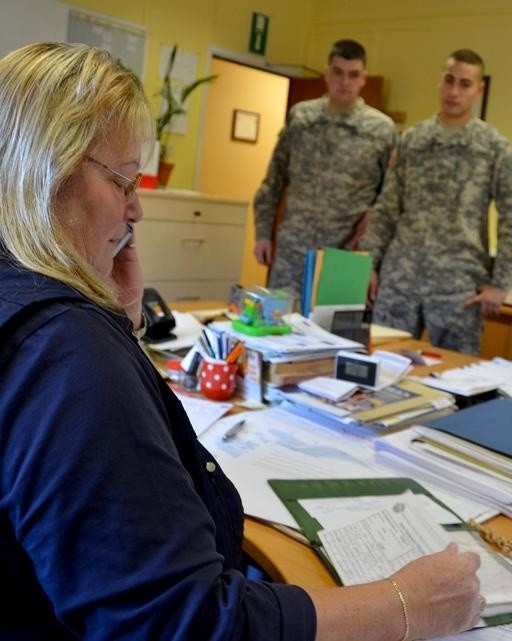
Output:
[81,154,145,198]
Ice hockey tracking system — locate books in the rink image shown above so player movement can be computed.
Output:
[210,247,512,521]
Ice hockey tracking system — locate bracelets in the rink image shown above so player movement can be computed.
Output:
[131,310,148,342]
[387,578,411,640]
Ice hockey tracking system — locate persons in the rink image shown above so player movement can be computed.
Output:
[251,39,398,310]
[352,47,512,358]
[1,40,487,638]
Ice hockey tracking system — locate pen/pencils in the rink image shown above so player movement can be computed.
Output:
[199,328,244,364]
[421,351,443,359]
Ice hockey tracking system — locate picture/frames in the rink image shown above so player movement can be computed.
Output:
[231,107,262,143]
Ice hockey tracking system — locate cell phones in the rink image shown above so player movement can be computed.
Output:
[112,222,134,259]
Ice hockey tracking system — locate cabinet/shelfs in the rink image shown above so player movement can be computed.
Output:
[126,188,249,311]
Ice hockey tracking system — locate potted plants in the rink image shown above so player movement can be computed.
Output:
[149,42,222,187]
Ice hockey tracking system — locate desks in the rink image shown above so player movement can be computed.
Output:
[131,300,510,641]
